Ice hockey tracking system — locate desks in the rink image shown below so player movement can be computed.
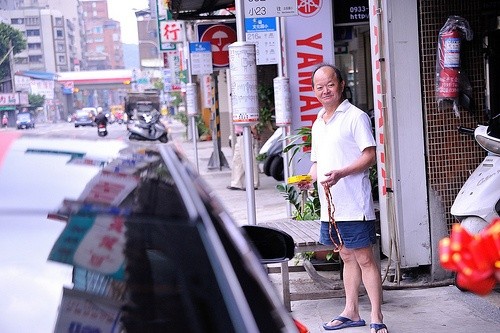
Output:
[268,219,385,313]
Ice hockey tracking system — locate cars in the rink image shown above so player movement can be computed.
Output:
[16,112,35,128]
[1,129,312,333]
[74,110,94,126]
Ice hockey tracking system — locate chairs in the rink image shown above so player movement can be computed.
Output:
[243,224,293,315]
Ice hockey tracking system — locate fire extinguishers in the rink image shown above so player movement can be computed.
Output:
[439,18,466,100]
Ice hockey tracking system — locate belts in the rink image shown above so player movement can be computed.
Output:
[236,131,253,136]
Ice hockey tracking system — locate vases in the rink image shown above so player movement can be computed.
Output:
[317,251,337,262]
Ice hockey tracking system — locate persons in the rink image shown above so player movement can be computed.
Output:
[2,115,8,129]
[297,64,388,333]
[94,107,109,135]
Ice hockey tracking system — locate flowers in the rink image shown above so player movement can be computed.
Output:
[275,175,321,265]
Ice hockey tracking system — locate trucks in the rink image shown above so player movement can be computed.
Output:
[124,88,162,124]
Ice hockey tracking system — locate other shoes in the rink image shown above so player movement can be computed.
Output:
[244,188,258,191]
[231,187,242,190]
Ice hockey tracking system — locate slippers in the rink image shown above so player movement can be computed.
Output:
[370,323,388,333]
[323,315,365,330]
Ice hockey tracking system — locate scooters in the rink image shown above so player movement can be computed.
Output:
[94,120,108,137]
[259,115,284,181]
[128,112,169,142]
[450,124,500,291]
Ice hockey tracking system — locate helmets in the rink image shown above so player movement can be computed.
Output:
[97,107,102,113]
